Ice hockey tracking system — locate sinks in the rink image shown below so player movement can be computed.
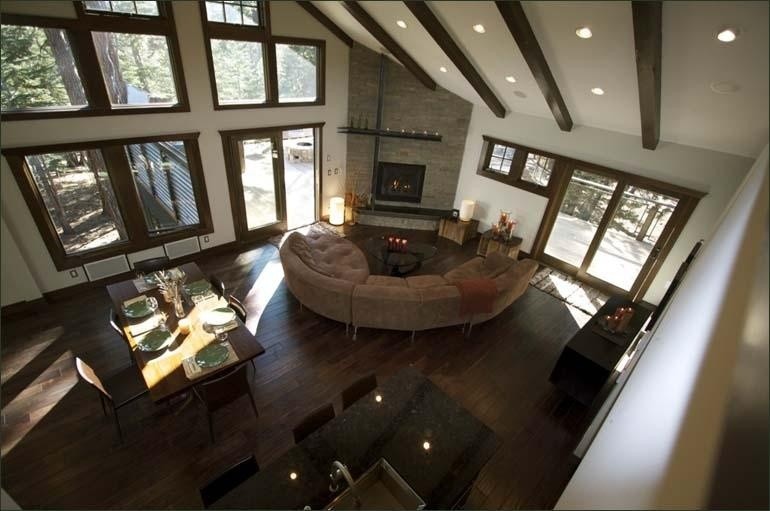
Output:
[323,457,426,511]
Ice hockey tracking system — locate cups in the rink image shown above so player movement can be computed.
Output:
[212,325,230,341]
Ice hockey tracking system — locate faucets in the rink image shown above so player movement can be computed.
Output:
[328,461,362,509]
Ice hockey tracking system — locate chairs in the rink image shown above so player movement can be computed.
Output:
[291,402,336,444]
[278,225,539,344]
[193,363,258,443]
[109,308,135,366]
[198,454,260,508]
[75,356,149,444]
[209,274,225,296]
[229,294,248,323]
[133,256,170,275]
[341,374,377,410]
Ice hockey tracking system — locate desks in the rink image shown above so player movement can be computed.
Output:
[286,144,314,161]
[438,215,480,246]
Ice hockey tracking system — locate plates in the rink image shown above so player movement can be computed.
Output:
[206,306,237,325]
[184,280,212,295]
[122,298,157,318]
[136,327,173,353]
[143,269,173,286]
[194,342,229,368]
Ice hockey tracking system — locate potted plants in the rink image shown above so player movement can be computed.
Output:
[155,269,186,318]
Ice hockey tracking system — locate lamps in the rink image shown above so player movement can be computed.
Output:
[459,200,475,221]
[330,197,344,226]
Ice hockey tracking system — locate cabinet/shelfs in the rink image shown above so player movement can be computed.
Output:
[548,295,652,408]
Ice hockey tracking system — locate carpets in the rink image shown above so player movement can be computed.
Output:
[528,267,611,317]
